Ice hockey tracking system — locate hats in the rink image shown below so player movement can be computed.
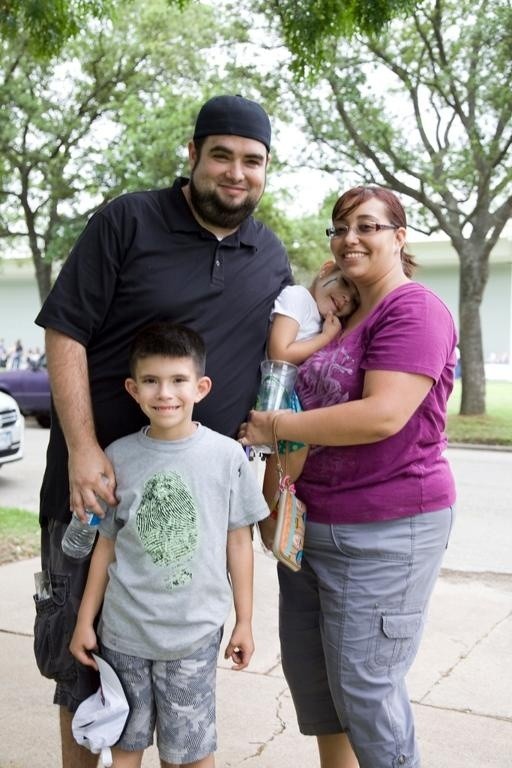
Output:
[72,652,130,766]
[194,95,271,152]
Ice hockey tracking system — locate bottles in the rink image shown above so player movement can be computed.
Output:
[60,476,112,559]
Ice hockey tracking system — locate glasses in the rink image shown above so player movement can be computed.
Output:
[326,223,396,237]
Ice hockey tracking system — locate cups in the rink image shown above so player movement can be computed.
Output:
[250,358,301,455]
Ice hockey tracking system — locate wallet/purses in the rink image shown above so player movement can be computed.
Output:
[272,489,306,572]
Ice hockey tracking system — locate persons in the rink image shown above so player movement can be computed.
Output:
[32,93,295,768]
[254,260,362,550]
[236,183,456,768]
[67,324,270,768]
[0,339,38,373]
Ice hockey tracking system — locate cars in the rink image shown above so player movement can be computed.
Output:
[0,392,24,468]
[0,350,52,428]
